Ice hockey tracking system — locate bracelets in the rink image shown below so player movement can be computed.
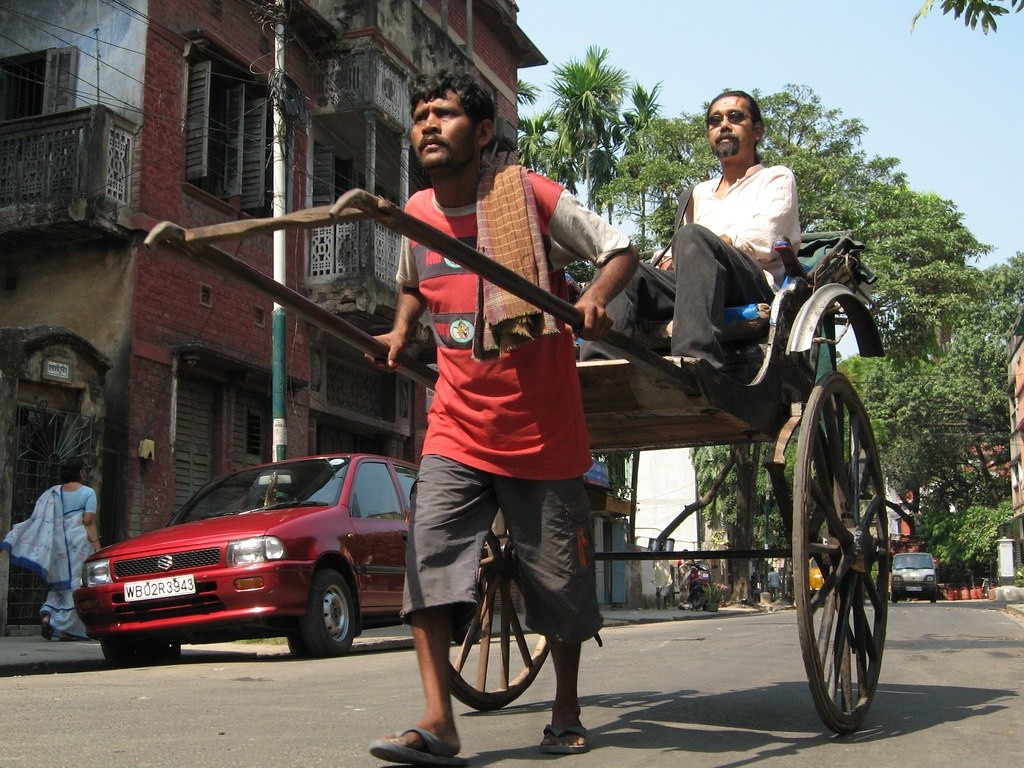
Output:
[91,541,96,543]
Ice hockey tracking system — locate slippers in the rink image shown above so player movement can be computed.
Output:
[60,635,79,641]
[539,724,590,754]
[41,623,51,640]
[369,728,468,768]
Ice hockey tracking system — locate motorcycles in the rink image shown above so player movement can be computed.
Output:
[682,549,719,612]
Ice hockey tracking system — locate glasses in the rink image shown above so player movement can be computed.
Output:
[707,111,752,125]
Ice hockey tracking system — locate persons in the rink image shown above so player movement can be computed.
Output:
[678,549,695,604]
[364,71,641,768]
[768,567,780,599]
[0,457,101,641]
[750,575,762,598]
[580,90,801,377]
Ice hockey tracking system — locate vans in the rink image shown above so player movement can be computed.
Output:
[888,553,939,604]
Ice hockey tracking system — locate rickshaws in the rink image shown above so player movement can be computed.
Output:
[142,189,894,736]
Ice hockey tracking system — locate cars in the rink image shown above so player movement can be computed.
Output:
[73,453,489,670]
